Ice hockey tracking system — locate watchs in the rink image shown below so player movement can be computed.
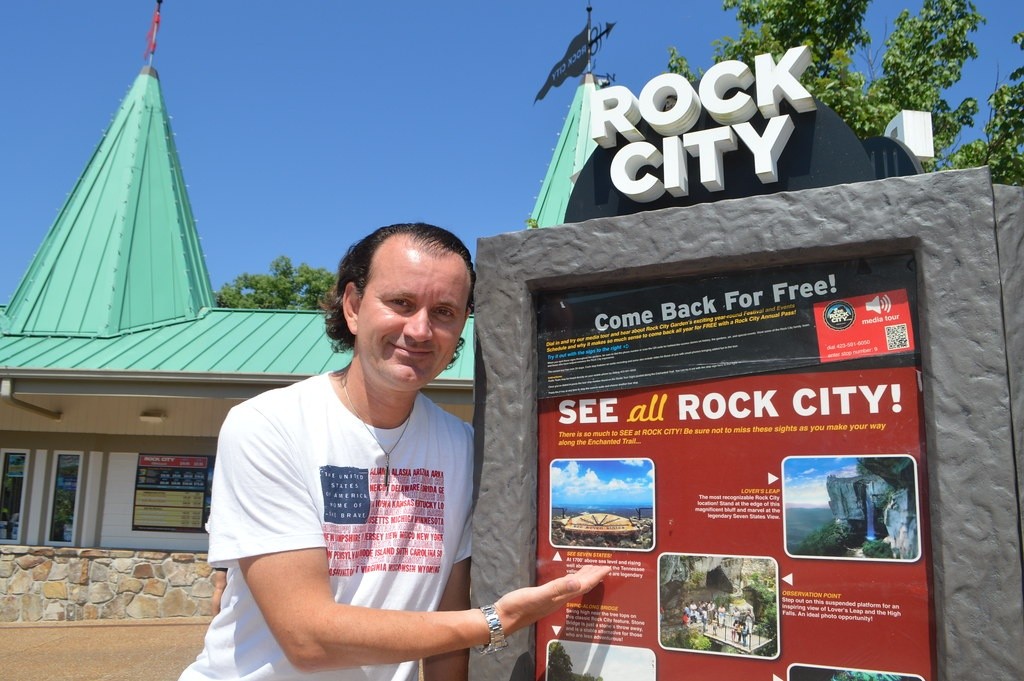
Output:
[475,604,508,655]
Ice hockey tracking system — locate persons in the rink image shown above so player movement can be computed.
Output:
[682,600,754,647]
[211,568,228,616]
[177,223,611,681]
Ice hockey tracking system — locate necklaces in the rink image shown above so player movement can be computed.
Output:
[342,376,411,486]
